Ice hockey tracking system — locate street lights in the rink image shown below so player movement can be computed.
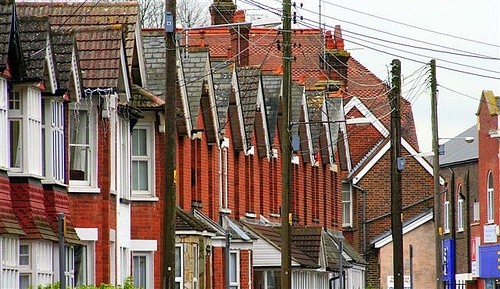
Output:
[389,152,436,289]
[432,136,475,289]
[162,20,253,288]
[281,117,371,289]
